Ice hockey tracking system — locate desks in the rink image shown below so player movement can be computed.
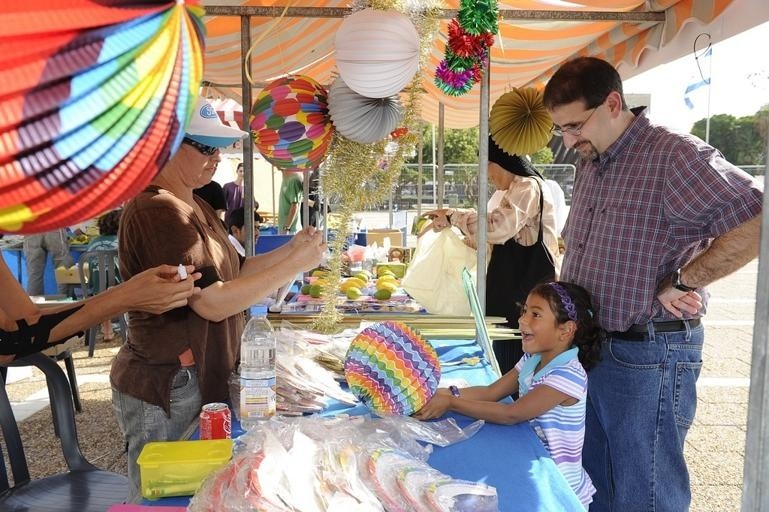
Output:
[139,255,594,512]
[1,233,85,297]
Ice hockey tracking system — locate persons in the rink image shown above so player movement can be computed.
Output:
[0,126,326,509]
[422,136,568,403]
[410,282,597,512]
[541,57,764,512]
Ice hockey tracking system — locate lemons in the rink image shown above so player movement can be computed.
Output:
[301,270,399,299]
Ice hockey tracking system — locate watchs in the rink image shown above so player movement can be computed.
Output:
[672,268,698,293]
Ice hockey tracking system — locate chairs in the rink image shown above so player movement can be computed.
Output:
[0,355,132,512]
[17,302,85,439]
[77,251,128,357]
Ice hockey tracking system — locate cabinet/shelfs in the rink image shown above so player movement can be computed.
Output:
[258,235,353,265]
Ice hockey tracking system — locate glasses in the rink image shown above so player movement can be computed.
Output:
[548,106,597,139]
[183,138,219,156]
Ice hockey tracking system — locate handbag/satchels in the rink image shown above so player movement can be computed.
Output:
[487,178,556,378]
[398,215,480,319]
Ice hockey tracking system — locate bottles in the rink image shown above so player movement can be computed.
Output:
[237,301,280,434]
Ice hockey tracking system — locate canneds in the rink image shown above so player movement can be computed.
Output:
[199,402,232,441]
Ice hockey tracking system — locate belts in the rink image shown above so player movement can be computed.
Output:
[603,316,703,342]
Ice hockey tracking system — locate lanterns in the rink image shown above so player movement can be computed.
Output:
[247,73,336,174]
[321,75,406,146]
[312,319,443,419]
[0,0,207,236]
[336,7,422,99]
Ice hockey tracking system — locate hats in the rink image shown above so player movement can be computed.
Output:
[184,96,250,149]
